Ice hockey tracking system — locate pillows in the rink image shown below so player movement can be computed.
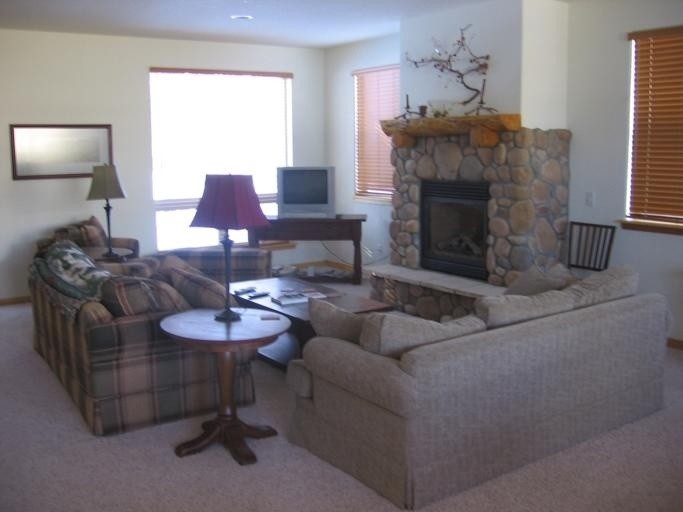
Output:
[304,295,363,345]
[501,260,578,297]
[43,217,241,316]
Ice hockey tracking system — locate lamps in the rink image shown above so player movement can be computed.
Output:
[82,162,126,259]
[189,172,274,323]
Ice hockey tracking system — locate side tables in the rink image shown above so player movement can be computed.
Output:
[155,306,292,464]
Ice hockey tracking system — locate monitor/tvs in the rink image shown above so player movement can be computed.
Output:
[277,166,336,219]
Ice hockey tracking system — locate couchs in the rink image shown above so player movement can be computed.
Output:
[285,267,669,512]
[25,214,272,436]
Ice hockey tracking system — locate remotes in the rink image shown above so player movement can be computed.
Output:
[248,290,270,298]
[234,287,255,294]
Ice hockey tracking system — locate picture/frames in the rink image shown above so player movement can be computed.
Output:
[7,121,114,182]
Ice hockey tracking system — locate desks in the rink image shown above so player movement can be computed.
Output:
[244,213,369,284]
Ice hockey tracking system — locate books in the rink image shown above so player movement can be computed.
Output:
[270,290,328,305]
